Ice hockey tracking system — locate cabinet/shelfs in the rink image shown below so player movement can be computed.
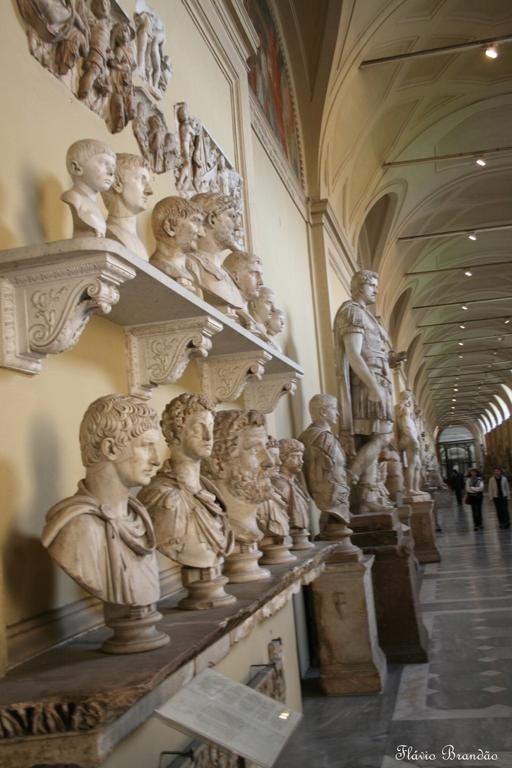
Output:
[1,230,350,768]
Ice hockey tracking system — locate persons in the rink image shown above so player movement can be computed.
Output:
[449,471,464,505]
[489,466,511,529]
[465,469,485,532]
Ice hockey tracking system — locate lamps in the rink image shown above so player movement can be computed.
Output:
[483,40,504,61]
[473,150,489,168]
[466,229,479,242]
